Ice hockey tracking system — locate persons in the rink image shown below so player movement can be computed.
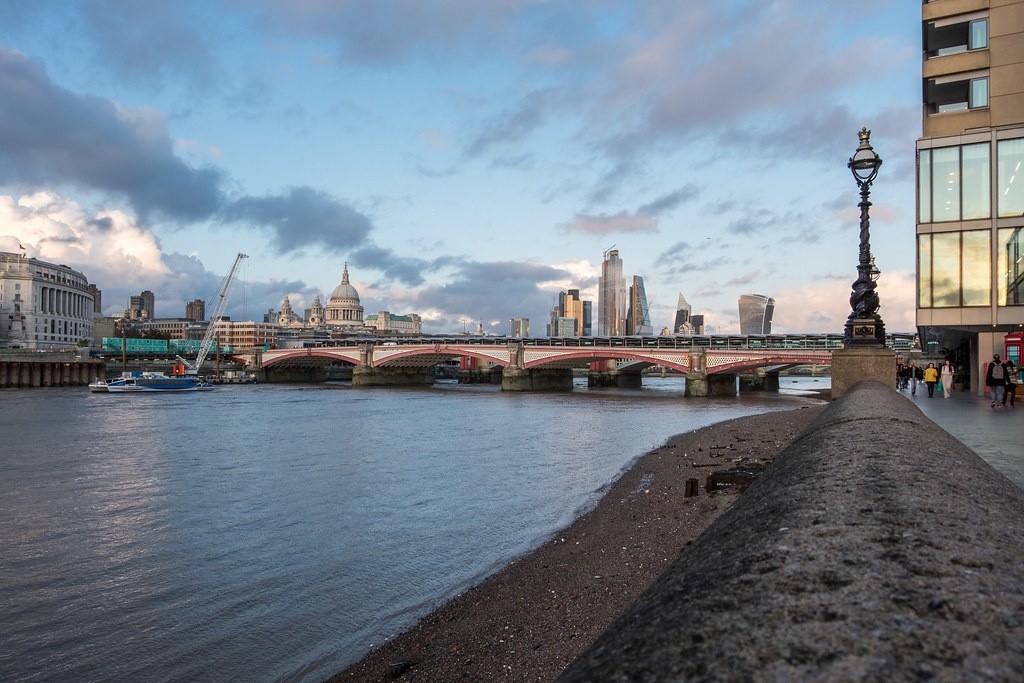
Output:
[898,360,955,398]
[985,354,1018,408]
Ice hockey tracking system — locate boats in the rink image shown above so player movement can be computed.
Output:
[434,378,458,384]
[88,371,215,391]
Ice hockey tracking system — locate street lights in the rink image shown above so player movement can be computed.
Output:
[463,319,467,344]
[122,327,126,371]
[841,127,887,349]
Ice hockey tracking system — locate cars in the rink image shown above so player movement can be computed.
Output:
[383,341,397,345]
[37,348,53,353]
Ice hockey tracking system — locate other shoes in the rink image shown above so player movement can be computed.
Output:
[997,404,1002,408]
[947,395,950,397]
[990,402,995,407]
[945,397,947,398]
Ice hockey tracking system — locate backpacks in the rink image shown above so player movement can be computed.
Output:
[991,362,1003,379]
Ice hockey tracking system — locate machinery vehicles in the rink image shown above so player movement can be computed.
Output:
[169,252,249,378]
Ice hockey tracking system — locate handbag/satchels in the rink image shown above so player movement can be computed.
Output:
[937,380,943,392]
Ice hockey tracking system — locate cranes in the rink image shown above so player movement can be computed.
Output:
[602,245,615,335]
